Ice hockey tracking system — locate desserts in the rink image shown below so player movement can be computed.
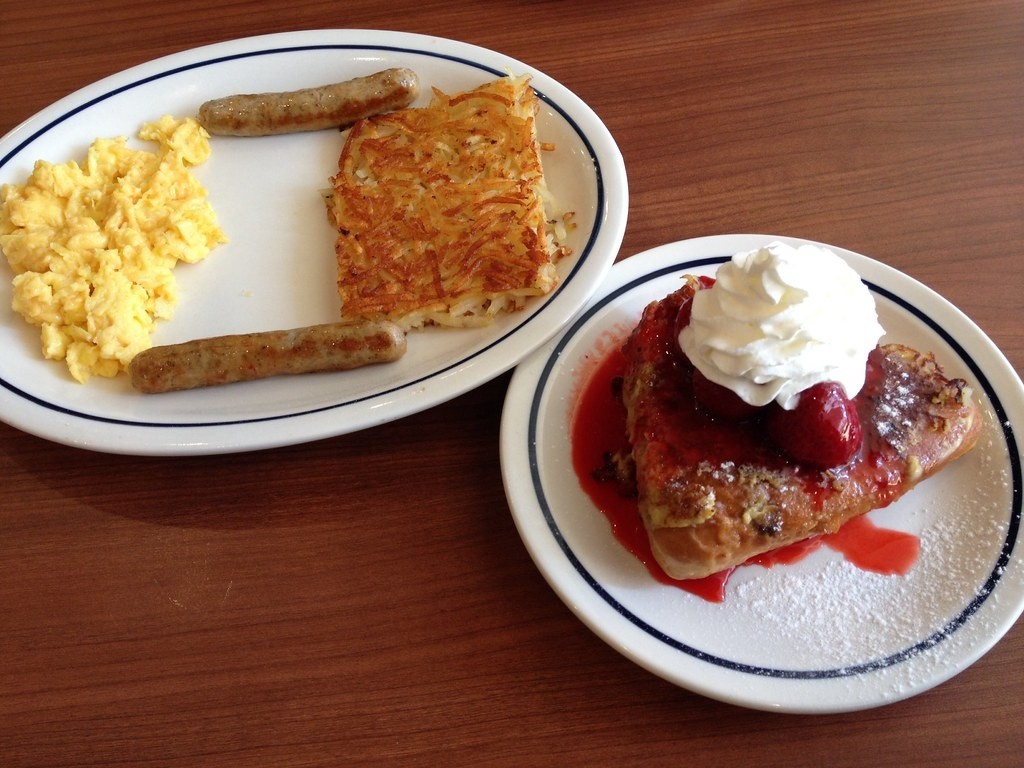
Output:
[624,240,982,581]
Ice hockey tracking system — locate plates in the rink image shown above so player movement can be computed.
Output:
[0,30,630,452]
[500,234,1024,714]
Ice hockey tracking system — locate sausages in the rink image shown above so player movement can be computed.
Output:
[198,68,421,138]
[130,319,406,395]
